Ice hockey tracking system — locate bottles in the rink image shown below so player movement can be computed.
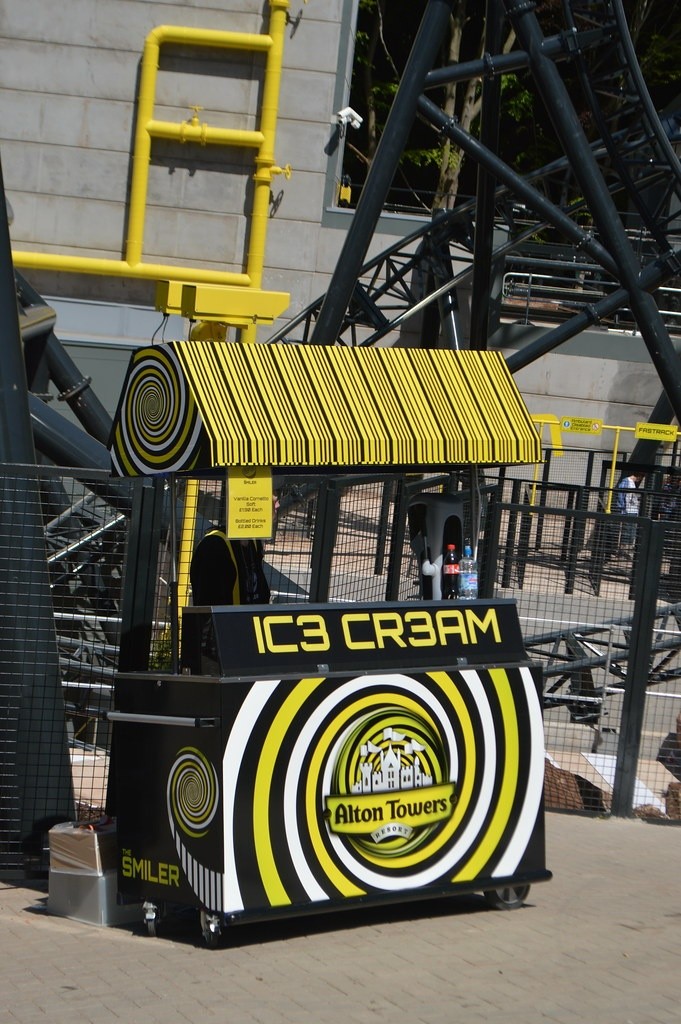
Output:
[456,545,479,600]
[442,544,460,599]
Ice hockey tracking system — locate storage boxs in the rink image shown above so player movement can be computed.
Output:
[49,821,112,876]
[48,870,143,927]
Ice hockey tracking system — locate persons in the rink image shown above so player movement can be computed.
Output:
[616,471,641,549]
[658,474,681,555]
[191,476,283,606]
